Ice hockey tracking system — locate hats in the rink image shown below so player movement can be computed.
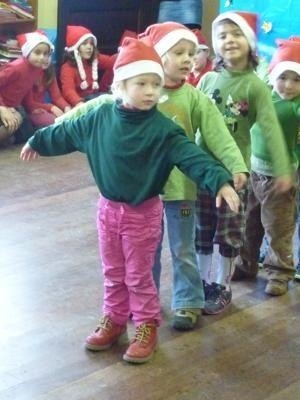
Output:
[16,33,50,58]
[267,36,300,84]
[138,21,199,57]
[211,11,257,58]
[117,30,137,51]
[192,29,208,50]
[112,37,165,86]
[64,25,97,52]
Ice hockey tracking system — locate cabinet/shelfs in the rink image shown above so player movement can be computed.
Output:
[0,0,38,70]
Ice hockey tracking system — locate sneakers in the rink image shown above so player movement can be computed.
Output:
[84,316,126,351]
[123,322,159,363]
[202,282,232,314]
[264,276,294,295]
[231,265,256,281]
[172,308,202,330]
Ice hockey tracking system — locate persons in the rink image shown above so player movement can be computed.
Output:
[197,12,290,315]
[232,37,300,295]
[59,26,118,106]
[49,22,249,331]
[23,49,72,128]
[100,30,140,94]
[19,36,242,364]
[0,29,57,146]
[190,27,212,88]
